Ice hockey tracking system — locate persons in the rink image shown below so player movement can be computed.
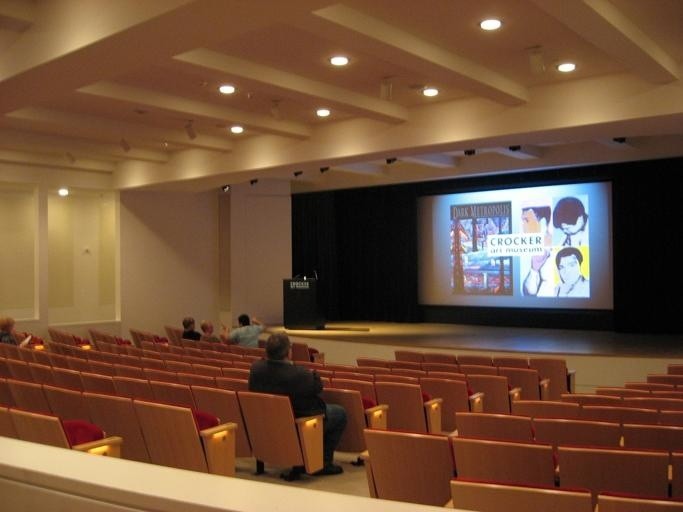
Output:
[522,247,589,299]
[182,317,201,341]
[551,196,588,246]
[520,206,551,247]
[0,316,32,346]
[247,332,347,482]
[217,314,266,345]
[199,319,220,343]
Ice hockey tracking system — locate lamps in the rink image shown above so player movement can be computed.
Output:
[508,145,521,152]
[293,171,303,177]
[463,148,476,156]
[613,136,627,144]
[320,166,329,174]
[221,185,232,193]
[249,179,259,185]
[385,157,397,164]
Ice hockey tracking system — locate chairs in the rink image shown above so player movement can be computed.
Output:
[1,328,682,512]
